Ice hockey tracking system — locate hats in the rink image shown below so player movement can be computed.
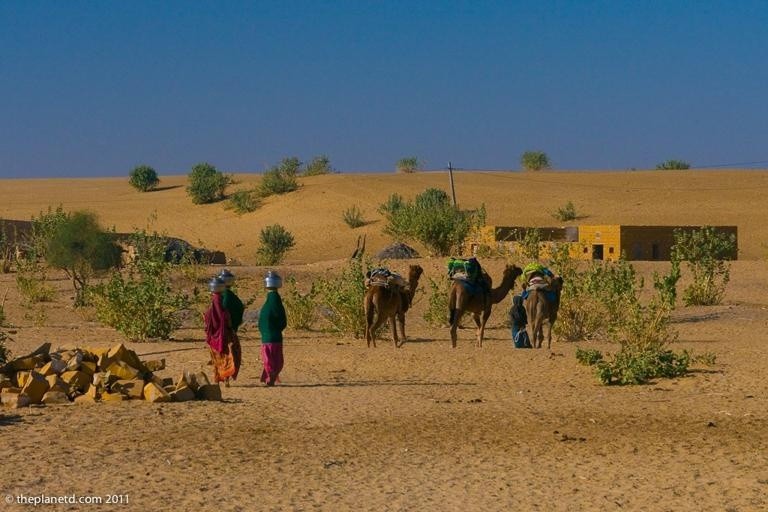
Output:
[209,272,235,291]
[264,271,282,288]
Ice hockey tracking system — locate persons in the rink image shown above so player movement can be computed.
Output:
[256,287,288,388]
[509,295,531,349]
[203,293,242,386]
[213,286,245,332]
